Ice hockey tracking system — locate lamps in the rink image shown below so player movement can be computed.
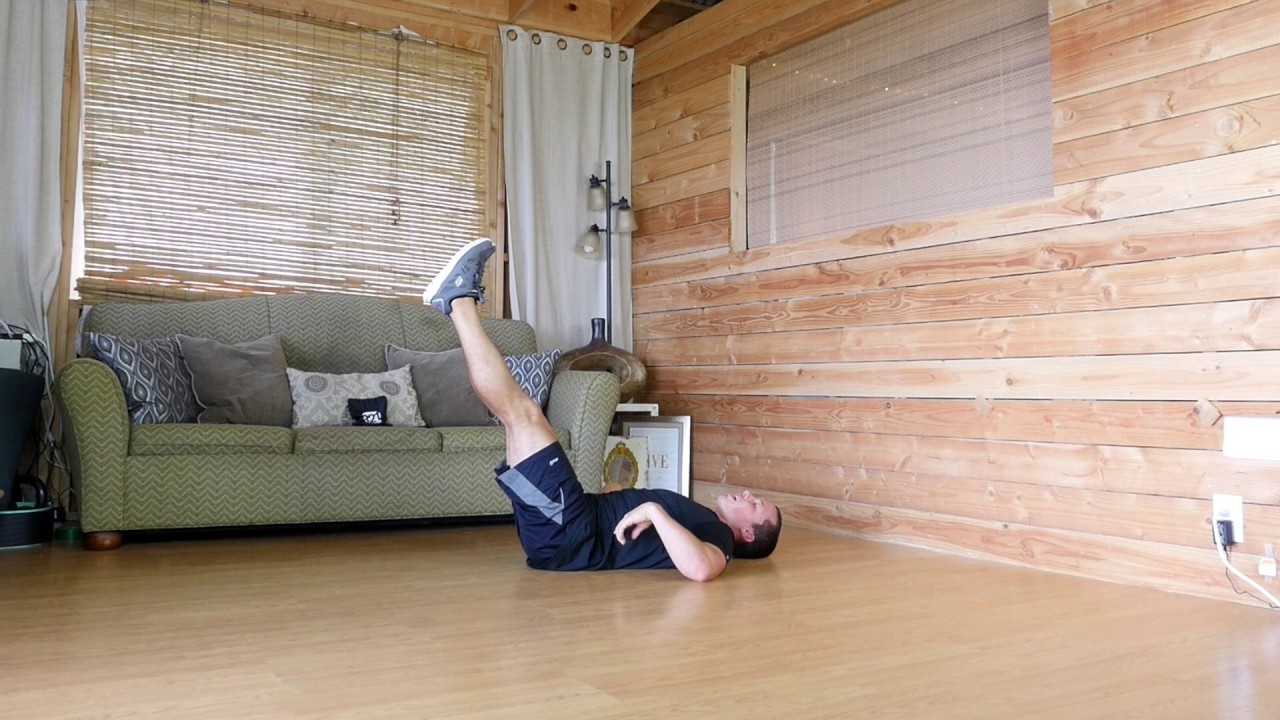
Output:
[573,160,640,348]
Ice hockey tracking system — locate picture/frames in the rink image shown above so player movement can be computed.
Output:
[600,435,650,492]
[615,414,693,503]
[608,402,660,435]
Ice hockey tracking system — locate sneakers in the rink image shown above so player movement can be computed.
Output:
[420,236,496,316]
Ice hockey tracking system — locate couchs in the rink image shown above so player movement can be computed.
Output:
[50,291,622,553]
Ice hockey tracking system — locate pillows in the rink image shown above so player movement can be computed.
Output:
[82,329,204,426]
[491,347,565,427]
[284,362,427,429]
[173,333,295,429]
[384,342,500,429]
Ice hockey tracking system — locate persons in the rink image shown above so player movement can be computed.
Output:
[422,238,782,582]
